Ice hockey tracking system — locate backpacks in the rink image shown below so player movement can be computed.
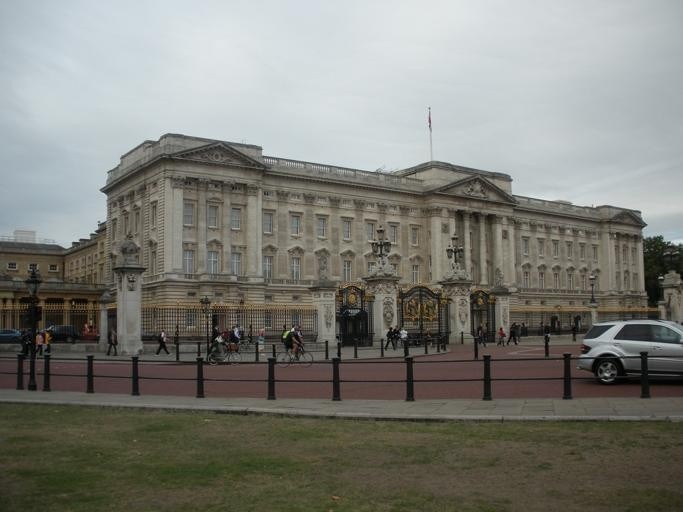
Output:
[282,330,291,343]
[158,334,163,343]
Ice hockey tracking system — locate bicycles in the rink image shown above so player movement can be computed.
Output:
[276,344,313,367]
[207,347,242,365]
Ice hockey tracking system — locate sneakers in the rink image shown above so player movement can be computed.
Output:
[291,356,298,360]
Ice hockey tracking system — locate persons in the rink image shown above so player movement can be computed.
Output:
[258,326,266,353]
[154,328,173,357]
[572,327,577,343]
[106,324,118,357]
[81,318,96,337]
[425,330,433,347]
[475,320,521,347]
[281,323,305,362]
[34,329,54,357]
[384,326,408,352]
[247,324,252,344]
[210,321,241,358]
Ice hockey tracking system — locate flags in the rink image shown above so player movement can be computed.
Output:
[429,109,433,132]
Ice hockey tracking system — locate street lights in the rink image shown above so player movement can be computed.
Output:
[199,295,211,355]
[364,225,402,282]
[445,233,464,281]
[657,273,665,301]
[588,275,597,303]
[25,266,43,390]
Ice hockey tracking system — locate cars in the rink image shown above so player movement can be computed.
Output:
[0,329,21,342]
[578,319,683,385]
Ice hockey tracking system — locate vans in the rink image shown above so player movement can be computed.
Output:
[40,325,74,343]
